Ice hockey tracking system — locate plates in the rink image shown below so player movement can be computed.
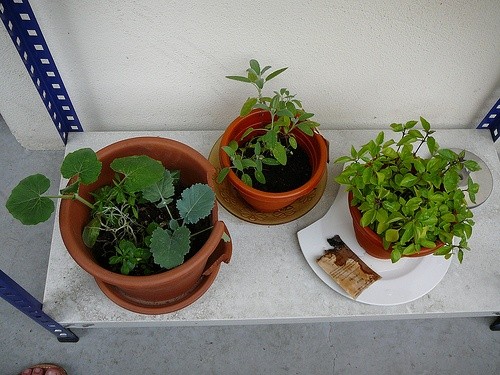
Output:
[297,159,455,306]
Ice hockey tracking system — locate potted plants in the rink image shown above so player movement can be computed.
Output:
[206,59,330,227]
[330,115,481,265]
[5,136,233,316]
